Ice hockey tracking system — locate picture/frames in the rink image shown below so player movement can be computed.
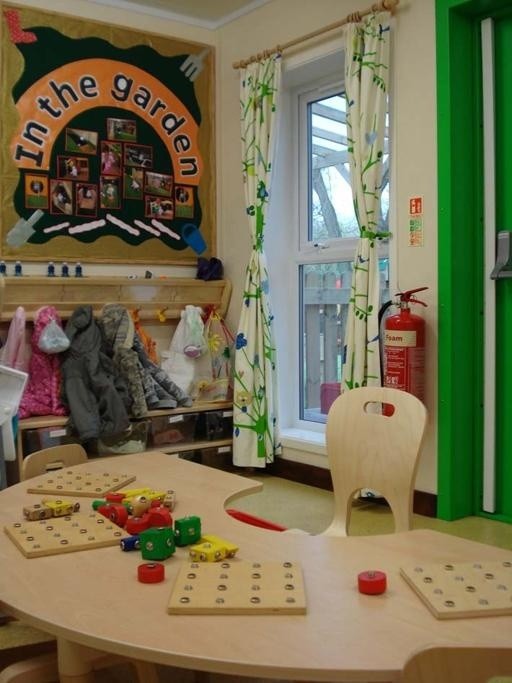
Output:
[0,0,219,266]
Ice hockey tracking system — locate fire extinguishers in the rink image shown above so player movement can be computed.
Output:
[379,287,429,416]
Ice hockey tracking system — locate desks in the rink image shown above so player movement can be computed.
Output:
[0,446,511,682]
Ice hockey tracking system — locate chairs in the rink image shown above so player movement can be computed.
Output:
[18,442,88,485]
[281,386,430,538]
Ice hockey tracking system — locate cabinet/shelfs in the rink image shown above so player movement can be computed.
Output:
[0,271,234,490]
